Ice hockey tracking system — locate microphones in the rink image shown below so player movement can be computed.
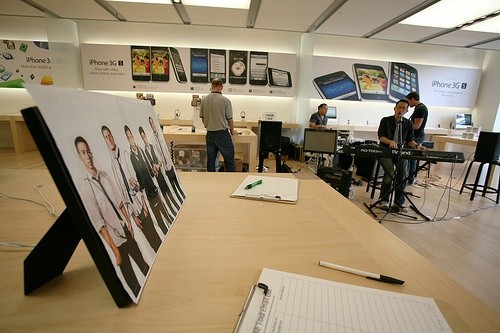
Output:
[399,114,403,122]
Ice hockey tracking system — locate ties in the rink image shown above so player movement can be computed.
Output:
[155,132,167,164]
[91,175,131,238]
[392,121,401,154]
[114,148,134,204]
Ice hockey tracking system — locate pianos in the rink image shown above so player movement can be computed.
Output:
[343,144,465,221]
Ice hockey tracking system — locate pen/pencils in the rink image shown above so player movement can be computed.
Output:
[245,180,262,189]
[319,260,405,285]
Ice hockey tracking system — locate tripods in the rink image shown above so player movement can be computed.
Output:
[370,122,418,225]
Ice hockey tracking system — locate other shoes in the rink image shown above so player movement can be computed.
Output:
[394,202,408,211]
[378,198,389,209]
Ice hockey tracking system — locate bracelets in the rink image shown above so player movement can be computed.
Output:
[416,143,422,149]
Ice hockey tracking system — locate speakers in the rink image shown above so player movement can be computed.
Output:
[356,154,384,179]
[474,131,500,161]
[258,119,282,150]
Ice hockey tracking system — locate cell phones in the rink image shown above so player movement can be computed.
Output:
[168,46,188,84]
[190,48,209,83]
[250,51,267,84]
[269,67,292,87]
[352,63,394,103]
[150,47,170,81]
[208,48,226,84]
[130,45,151,81]
[311,71,361,101]
[387,62,419,103]
[229,50,248,84]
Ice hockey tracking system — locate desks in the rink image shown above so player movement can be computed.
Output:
[329,125,496,186]
[0,115,30,154]
[164,126,258,174]
[0,169,500,333]
[157,117,299,130]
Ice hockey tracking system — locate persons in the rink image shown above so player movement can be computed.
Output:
[199,78,239,172]
[378,99,428,211]
[309,103,328,129]
[76,116,187,299]
[406,92,429,185]
